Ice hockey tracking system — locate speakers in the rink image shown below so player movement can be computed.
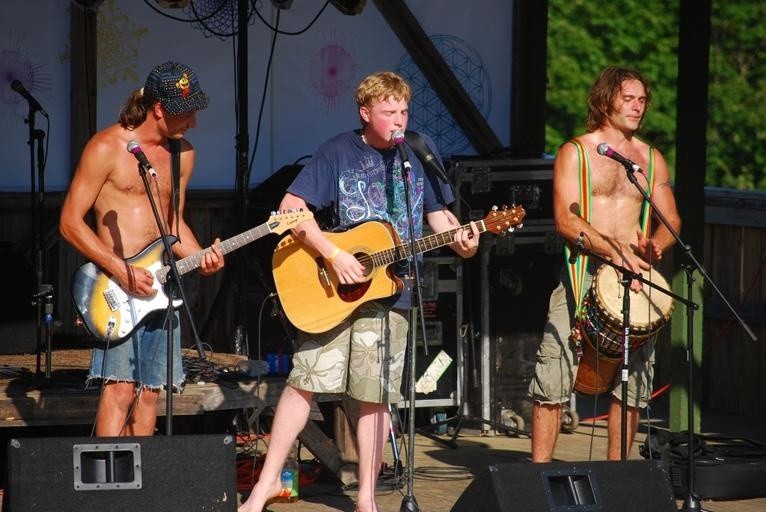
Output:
[6,432,240,511]
[449,459,681,511]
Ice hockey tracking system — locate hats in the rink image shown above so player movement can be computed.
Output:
[142,60,210,116]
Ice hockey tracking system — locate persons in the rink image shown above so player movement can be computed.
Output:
[235,72,481,512]
[60,61,224,437]
[527,66,681,463]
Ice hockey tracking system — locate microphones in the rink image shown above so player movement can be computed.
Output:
[597,143,644,174]
[391,129,412,174]
[10,80,48,118]
[569,232,585,264]
[126,140,156,177]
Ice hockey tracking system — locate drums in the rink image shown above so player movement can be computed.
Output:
[570,255,675,395]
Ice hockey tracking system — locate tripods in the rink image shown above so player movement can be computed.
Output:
[418,212,533,450]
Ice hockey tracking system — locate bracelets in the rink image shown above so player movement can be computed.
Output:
[327,247,340,264]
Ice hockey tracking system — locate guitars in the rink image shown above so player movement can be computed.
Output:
[269,203,527,337]
[71,208,314,348]
